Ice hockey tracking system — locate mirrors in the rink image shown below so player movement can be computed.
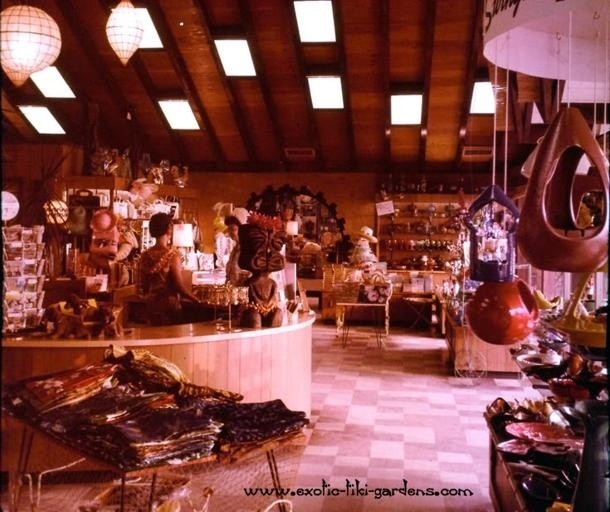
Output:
[243,183,352,280]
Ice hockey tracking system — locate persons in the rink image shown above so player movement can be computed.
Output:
[224,213,250,286]
[135,211,201,326]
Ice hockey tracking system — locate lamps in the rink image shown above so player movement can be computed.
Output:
[284,221,299,250]
[0,0,62,88]
[172,223,194,272]
[105,0,143,70]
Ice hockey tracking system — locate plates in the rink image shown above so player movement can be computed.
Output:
[517,353,547,365]
[505,421,573,444]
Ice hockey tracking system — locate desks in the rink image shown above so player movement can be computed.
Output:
[0,358,310,512]
[482,401,584,512]
[336,303,386,351]
[403,297,434,328]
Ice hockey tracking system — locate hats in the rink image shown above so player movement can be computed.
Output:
[353,225,379,244]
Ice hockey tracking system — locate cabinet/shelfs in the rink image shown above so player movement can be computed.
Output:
[375,201,465,275]
[507,310,610,435]
